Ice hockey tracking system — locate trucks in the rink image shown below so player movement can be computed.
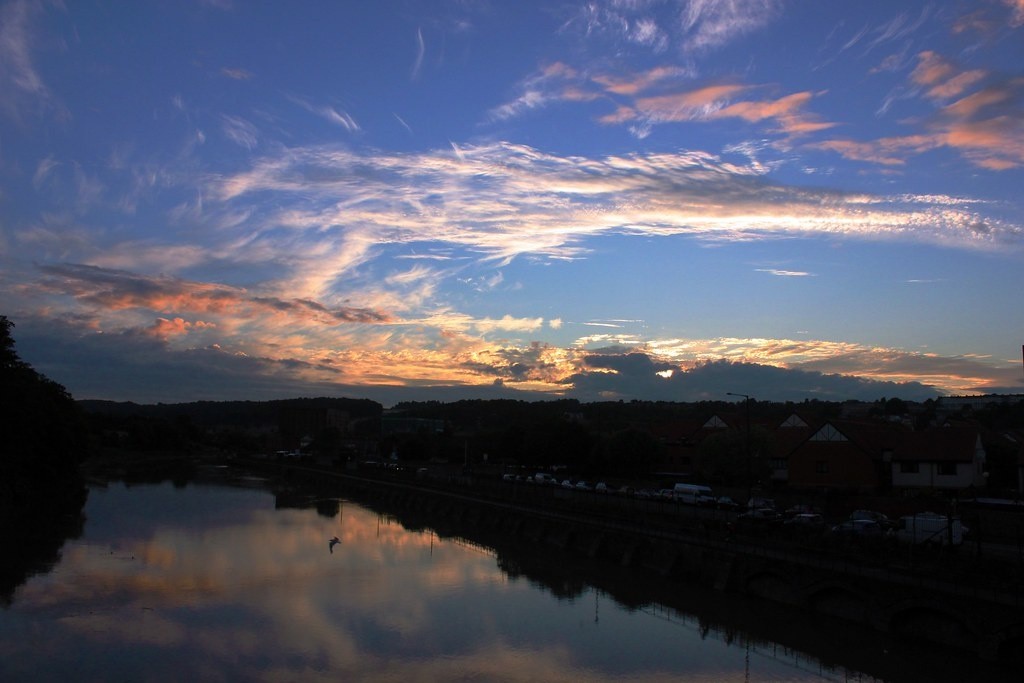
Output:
[673,482,717,508]
[886,515,963,548]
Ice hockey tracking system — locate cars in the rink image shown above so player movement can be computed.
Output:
[365,461,416,473]
[501,473,745,512]
[821,519,881,542]
[736,498,823,531]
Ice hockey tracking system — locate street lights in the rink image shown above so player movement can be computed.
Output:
[725,392,750,496]
